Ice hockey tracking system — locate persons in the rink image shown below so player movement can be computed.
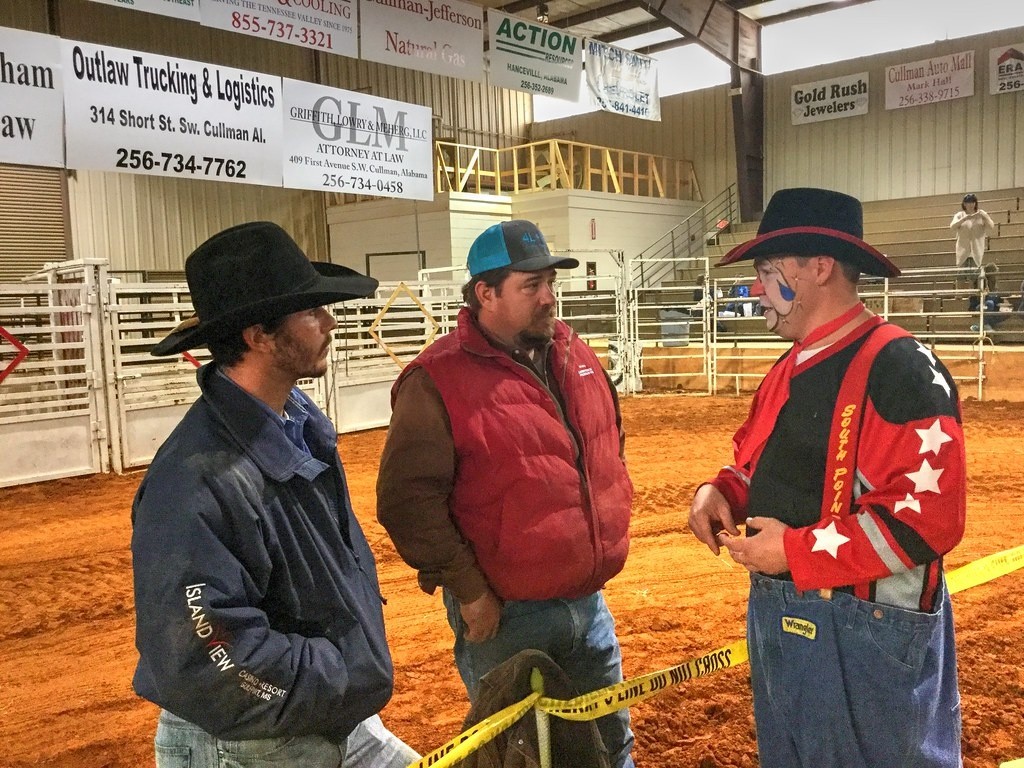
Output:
[128,219,422,768]
[950,194,994,300]
[687,185,971,766]
[375,219,634,768]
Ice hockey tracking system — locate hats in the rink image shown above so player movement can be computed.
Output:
[713,187,902,278]
[466,218,580,277]
[149,220,382,357]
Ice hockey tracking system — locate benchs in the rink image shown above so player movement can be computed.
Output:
[610,185,1024,344]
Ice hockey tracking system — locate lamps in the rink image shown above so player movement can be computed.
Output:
[535,1,548,24]
